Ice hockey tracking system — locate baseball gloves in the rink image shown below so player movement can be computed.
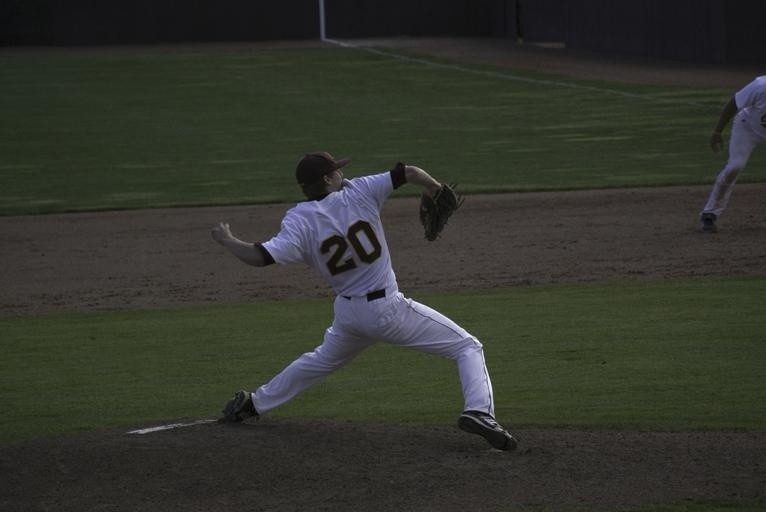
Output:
[420,181,465,242]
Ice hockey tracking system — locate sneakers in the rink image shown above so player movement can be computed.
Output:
[223,390,258,422]
[457,411,518,451]
[701,213,717,232]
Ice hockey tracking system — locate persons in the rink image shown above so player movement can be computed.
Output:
[697,74,766,233]
[210,149,517,454]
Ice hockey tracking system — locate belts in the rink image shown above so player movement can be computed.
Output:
[343,289,385,301]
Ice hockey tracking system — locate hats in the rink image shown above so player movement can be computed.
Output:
[296,152,350,184]
